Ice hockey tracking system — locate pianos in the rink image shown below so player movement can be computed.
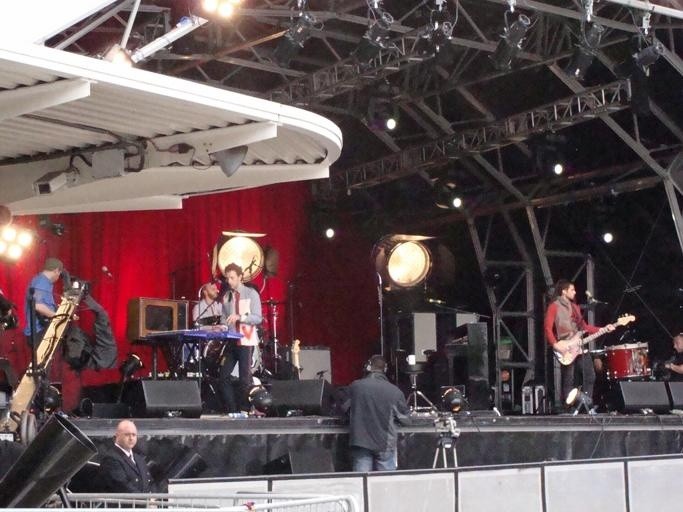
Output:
[150,330,244,340]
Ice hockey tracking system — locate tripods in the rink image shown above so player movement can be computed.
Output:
[406,375,438,411]
[432,438,458,468]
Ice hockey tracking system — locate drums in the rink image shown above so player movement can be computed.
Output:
[607,342,651,379]
[591,348,607,377]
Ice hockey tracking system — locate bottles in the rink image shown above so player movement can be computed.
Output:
[229,412,248,419]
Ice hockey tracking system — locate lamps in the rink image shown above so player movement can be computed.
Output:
[280,0,319,50]
[32,382,63,431]
[1,223,36,265]
[247,383,280,420]
[566,387,598,418]
[441,386,462,426]
[94,42,137,69]
[562,0,604,84]
[404,0,452,80]
[345,1,395,67]
[111,353,144,403]
[483,2,532,74]
[613,0,665,81]
[208,230,264,287]
[372,234,434,422]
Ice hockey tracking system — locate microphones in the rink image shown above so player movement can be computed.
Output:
[316,368,328,375]
[585,290,597,304]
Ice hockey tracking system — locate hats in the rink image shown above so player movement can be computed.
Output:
[41,257,64,272]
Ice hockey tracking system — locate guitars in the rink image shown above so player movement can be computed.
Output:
[554,313,636,366]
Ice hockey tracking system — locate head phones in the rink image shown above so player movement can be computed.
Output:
[364,355,389,373]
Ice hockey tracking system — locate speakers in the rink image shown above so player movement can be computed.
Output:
[286,346,332,385]
[272,380,336,416]
[129,379,202,417]
[608,381,671,414]
[397,313,437,362]
[666,382,683,410]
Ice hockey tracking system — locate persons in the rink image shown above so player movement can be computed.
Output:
[95,419,158,508]
[339,354,409,472]
[22,257,80,420]
[212,262,264,419]
[664,333,683,415]
[191,282,223,328]
[543,278,616,413]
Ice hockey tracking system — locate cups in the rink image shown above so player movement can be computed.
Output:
[405,354,415,365]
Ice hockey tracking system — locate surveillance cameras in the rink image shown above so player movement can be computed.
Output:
[32,172,67,196]
